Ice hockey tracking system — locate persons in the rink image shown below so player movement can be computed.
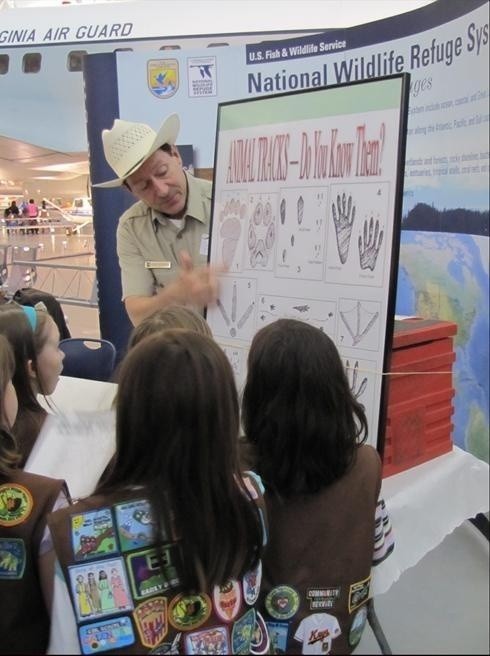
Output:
[0,302,68,478]
[127,304,215,353]
[0,336,81,656]
[75,569,128,617]
[47,328,270,655]
[236,318,395,656]
[5,200,51,235]
[92,114,230,327]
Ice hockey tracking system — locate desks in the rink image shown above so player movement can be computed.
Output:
[360,442,489,656]
[35,374,125,517]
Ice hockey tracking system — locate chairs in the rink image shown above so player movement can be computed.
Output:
[59,338,118,381]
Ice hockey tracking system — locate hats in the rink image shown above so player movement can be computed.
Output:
[90,114,181,188]
